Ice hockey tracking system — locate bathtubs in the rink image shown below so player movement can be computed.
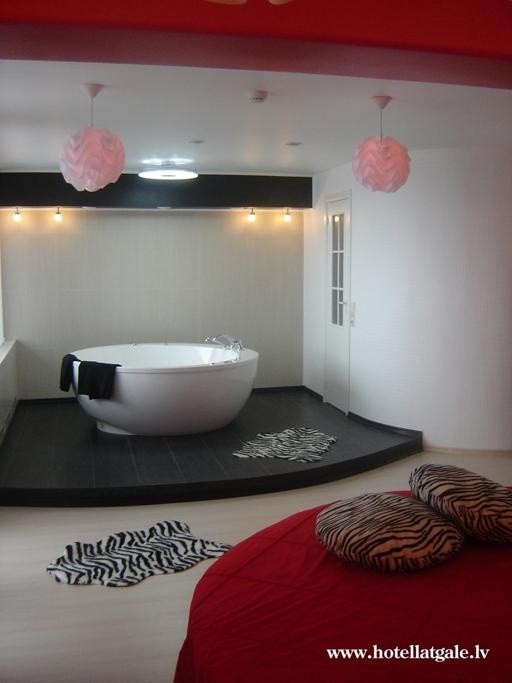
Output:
[72,343,260,435]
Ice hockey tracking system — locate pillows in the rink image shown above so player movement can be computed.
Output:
[315,488,464,572]
[408,462,512,544]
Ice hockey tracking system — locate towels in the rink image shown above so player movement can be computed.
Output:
[59,353,80,392]
[76,360,121,399]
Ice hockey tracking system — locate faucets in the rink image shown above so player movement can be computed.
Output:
[208,337,224,344]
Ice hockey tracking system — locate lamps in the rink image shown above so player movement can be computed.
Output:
[138,160,198,179]
[12,205,22,222]
[246,206,256,222]
[352,95,413,192]
[53,205,63,221]
[57,82,126,194]
[283,207,293,221]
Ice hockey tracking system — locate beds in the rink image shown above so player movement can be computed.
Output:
[173,490,512,680]
